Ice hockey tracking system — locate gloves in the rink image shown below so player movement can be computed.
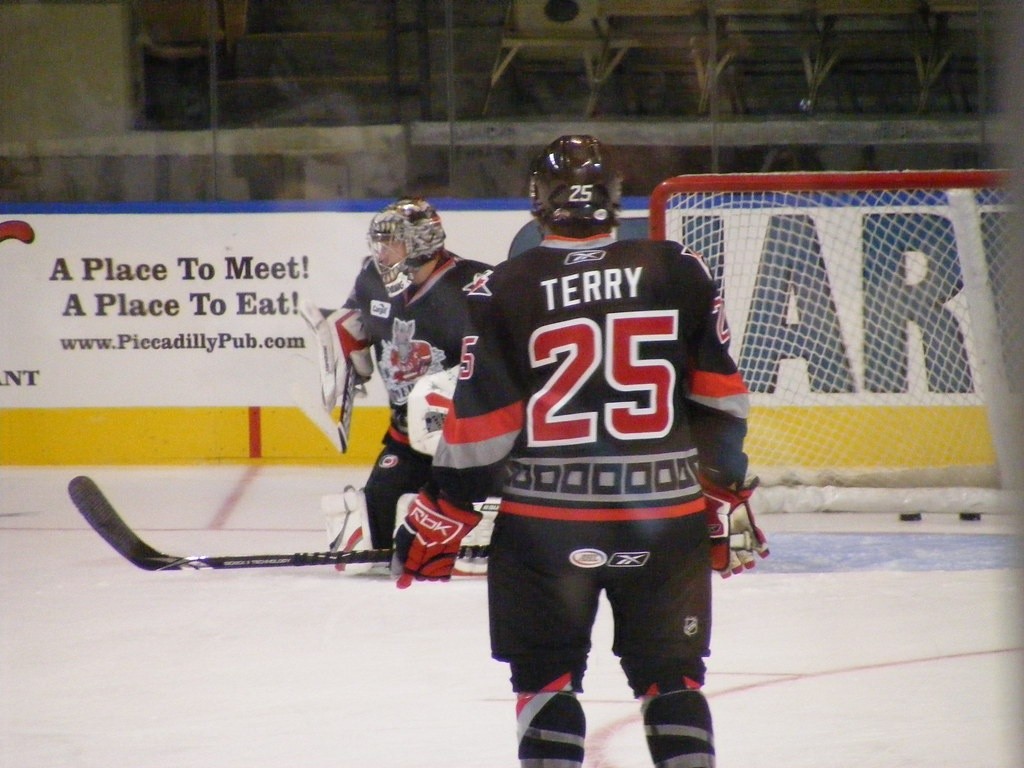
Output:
[698,470,770,579]
[395,482,483,589]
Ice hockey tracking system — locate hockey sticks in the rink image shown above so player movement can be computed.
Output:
[286,361,357,454]
[69,473,753,573]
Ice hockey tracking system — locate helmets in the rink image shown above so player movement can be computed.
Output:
[528,135,624,227]
[370,199,446,298]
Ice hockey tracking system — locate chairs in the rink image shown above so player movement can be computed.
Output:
[132,0,246,131]
[479,0,996,120]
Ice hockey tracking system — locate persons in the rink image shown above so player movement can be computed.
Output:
[300,200,497,571]
[391,133,769,768]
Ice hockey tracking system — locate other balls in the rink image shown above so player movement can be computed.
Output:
[900,512,922,522]
[958,511,982,521]
